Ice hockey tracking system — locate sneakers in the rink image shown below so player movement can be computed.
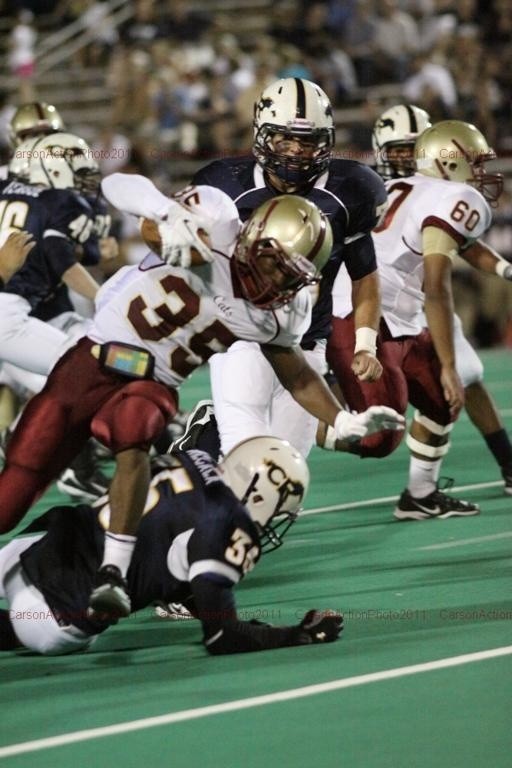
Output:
[393,487,484,523]
[166,397,218,458]
[58,465,110,503]
[84,566,136,628]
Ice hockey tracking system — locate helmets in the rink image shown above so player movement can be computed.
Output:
[369,103,432,179]
[8,98,103,202]
[251,77,336,189]
[230,192,335,312]
[415,118,503,204]
[221,431,308,556]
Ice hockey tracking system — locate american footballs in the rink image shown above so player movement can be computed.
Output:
[141,214,212,266]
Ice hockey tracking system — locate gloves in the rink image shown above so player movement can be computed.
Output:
[154,200,214,270]
[285,610,344,648]
[330,403,408,439]
